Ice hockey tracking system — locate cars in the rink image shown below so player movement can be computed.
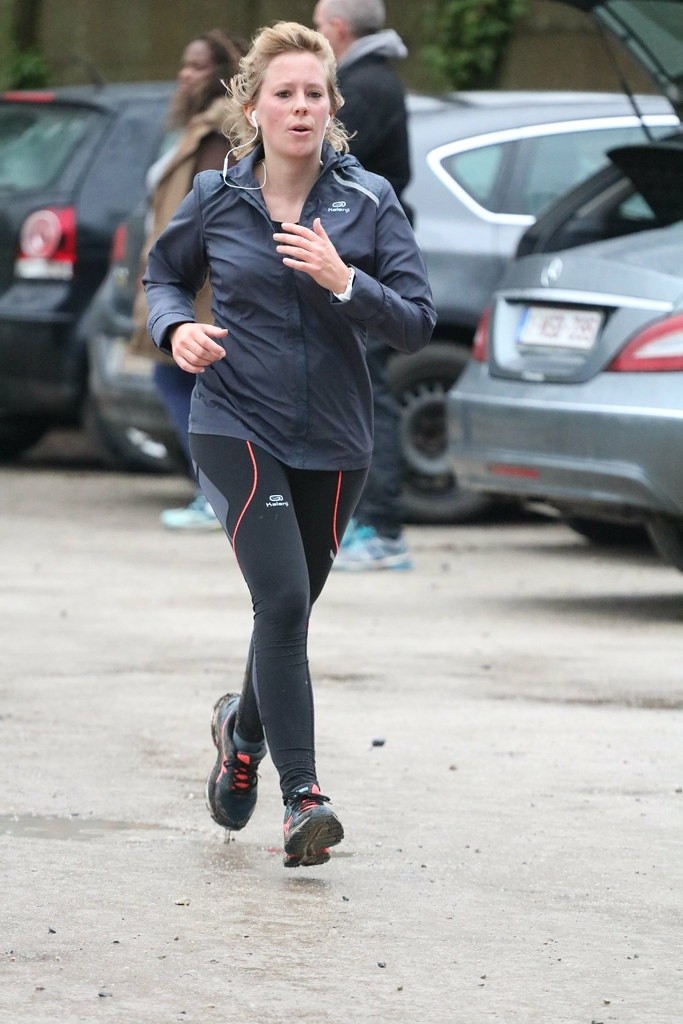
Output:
[80,88,683,525]
[0,87,175,477]
[444,142,683,578]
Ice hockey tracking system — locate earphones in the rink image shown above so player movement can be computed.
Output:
[252,110,260,129]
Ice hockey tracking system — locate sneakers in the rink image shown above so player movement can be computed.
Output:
[330,516,411,572]
[203,692,268,832]
[160,491,223,531]
[282,782,345,868]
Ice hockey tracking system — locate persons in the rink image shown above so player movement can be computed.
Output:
[128,28,244,529]
[141,20,440,868]
[311,0,413,569]
